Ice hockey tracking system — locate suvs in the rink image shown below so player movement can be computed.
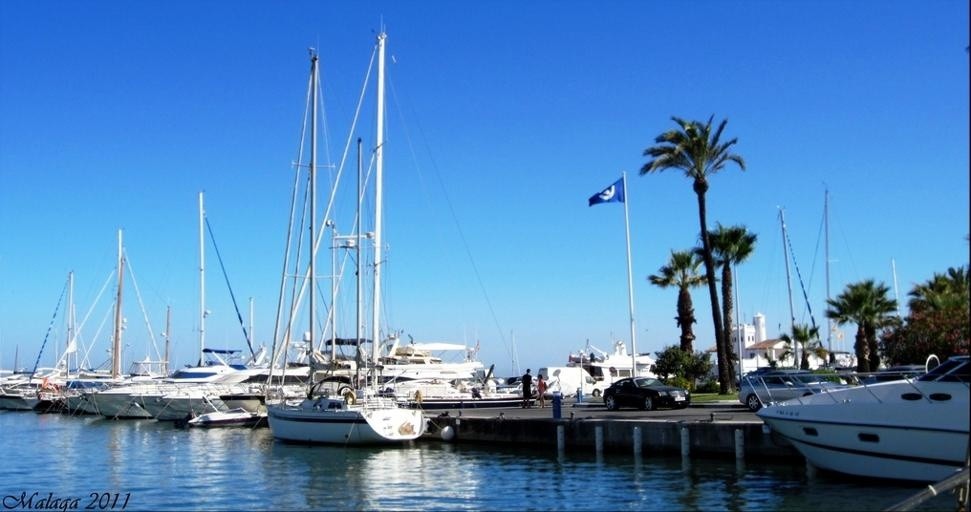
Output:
[736,367,852,412]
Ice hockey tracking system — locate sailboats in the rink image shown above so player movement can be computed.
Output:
[254,30,432,450]
[0,39,517,427]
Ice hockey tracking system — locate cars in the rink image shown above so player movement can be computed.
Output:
[602,376,693,411]
[861,364,929,386]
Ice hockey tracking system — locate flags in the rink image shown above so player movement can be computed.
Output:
[588,177,625,206]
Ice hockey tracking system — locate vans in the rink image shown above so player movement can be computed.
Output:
[536,364,605,398]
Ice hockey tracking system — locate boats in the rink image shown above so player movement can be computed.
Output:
[741,349,970,487]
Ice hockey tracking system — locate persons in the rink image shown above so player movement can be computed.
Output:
[538,373,545,409]
[522,369,532,409]
